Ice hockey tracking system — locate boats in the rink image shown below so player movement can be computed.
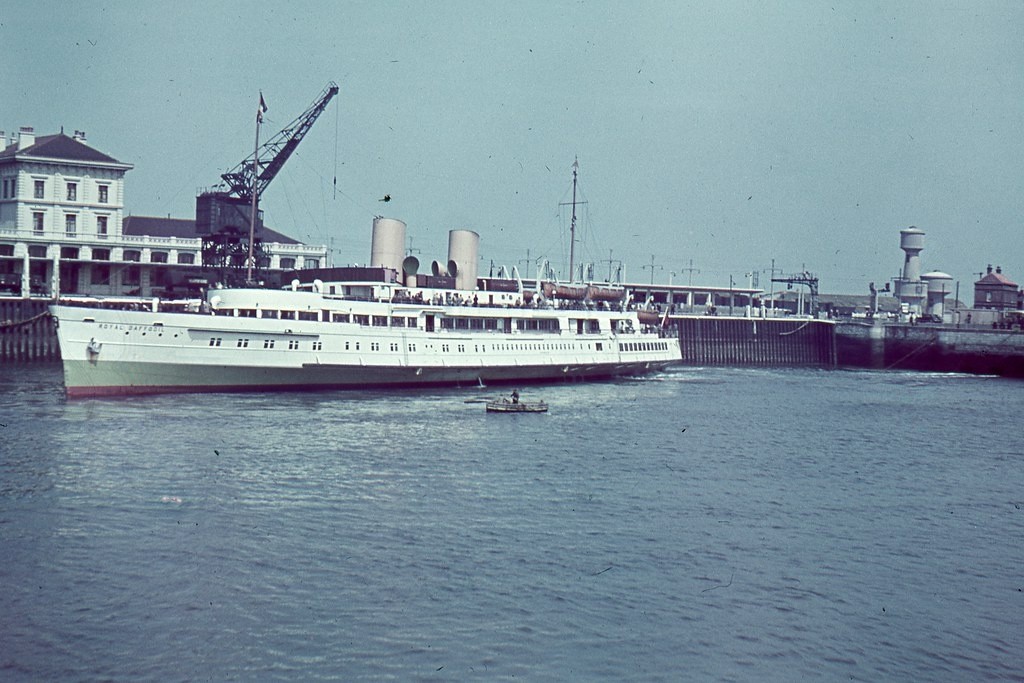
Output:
[47,81,684,399]
[486,399,549,413]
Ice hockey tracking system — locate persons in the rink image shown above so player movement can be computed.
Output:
[514,297,522,308]
[536,297,544,310]
[669,304,676,316]
[560,299,634,311]
[509,388,520,406]
[706,303,717,317]
[656,302,661,313]
[394,290,478,308]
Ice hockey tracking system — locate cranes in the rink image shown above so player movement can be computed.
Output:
[196,80,341,299]
